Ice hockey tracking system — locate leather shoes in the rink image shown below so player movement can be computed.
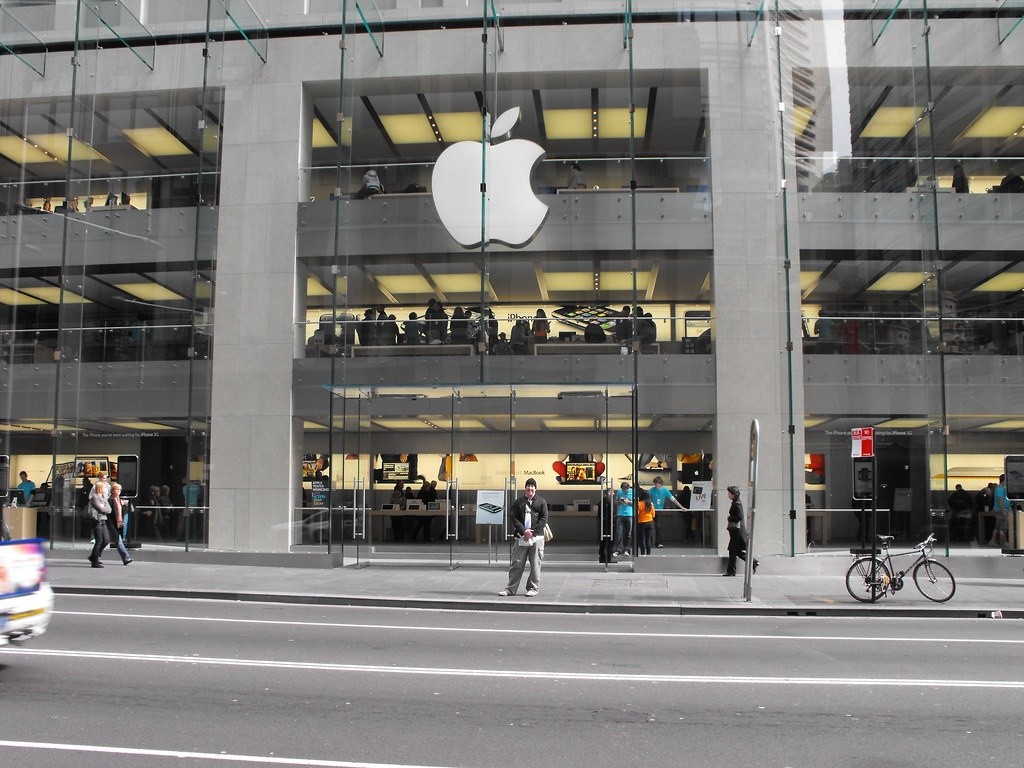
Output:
[723,571,736,576]
[753,561,760,575]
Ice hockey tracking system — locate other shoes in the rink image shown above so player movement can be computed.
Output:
[88,556,105,568]
[124,557,133,566]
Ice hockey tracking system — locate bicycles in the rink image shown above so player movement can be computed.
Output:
[845,532,956,602]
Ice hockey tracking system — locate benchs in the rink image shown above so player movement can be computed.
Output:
[351,344,477,356]
[557,188,681,194]
[368,192,432,199]
[533,343,660,356]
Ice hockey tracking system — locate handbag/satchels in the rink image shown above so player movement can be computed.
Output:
[88,508,102,528]
[541,522,554,542]
[727,520,741,531]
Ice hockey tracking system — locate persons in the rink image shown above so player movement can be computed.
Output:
[722,486,760,576]
[359,298,657,355]
[353,168,380,199]
[952,164,969,194]
[43,201,53,213]
[697,327,711,354]
[598,476,696,563]
[566,163,587,189]
[1000,168,1024,192]
[499,477,548,596]
[177,478,201,543]
[390,480,452,543]
[16,471,35,507]
[147,485,174,543]
[814,310,861,354]
[88,472,134,568]
[852,493,872,542]
[948,474,1010,547]
[71,200,79,213]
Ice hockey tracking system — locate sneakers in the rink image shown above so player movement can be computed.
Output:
[526,589,538,597]
[989,541,1000,547]
[624,551,629,557]
[613,552,619,556]
[657,544,663,549]
[608,559,617,563]
[499,590,508,596]
[1003,542,1010,547]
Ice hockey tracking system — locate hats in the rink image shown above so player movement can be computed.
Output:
[727,486,740,497]
[525,478,536,489]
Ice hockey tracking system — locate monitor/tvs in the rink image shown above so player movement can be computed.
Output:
[74,456,109,478]
[566,462,596,482]
[303,459,317,478]
[382,462,410,480]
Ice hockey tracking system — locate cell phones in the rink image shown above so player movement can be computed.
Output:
[1004,456,1024,501]
[0,455,10,497]
[551,306,634,335]
[117,456,138,498]
[685,311,711,338]
[852,454,877,500]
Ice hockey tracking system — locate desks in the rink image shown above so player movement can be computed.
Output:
[806,511,828,545]
[901,187,957,193]
[332,510,599,544]
[89,205,139,211]
[978,512,997,545]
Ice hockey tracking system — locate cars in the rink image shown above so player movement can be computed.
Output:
[0,539,56,646]
[275,508,364,544]
